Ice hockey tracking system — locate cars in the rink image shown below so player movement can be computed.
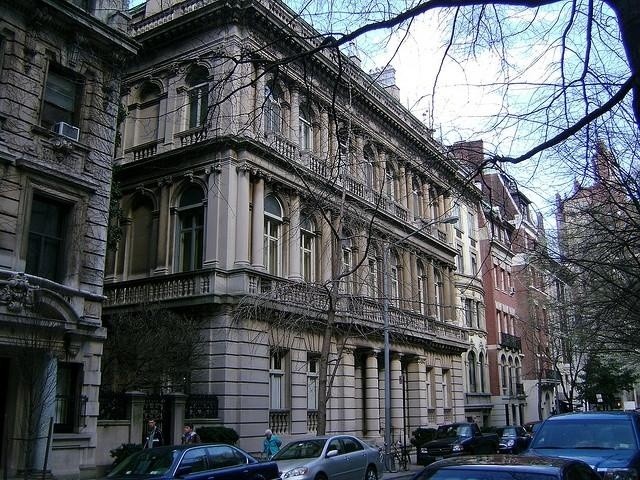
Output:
[412,408,639,480]
[270,434,385,480]
[102,443,279,480]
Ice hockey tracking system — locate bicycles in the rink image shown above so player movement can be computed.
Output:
[385,440,411,473]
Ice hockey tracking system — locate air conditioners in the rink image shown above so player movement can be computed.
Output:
[51,122,80,142]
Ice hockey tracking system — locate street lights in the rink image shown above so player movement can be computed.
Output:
[384,215,459,471]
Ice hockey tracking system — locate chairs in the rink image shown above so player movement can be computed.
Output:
[306,443,355,457]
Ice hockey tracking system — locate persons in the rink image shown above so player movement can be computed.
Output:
[551,407,557,417]
[143,419,166,448]
[182,423,201,444]
[263,428,282,459]
